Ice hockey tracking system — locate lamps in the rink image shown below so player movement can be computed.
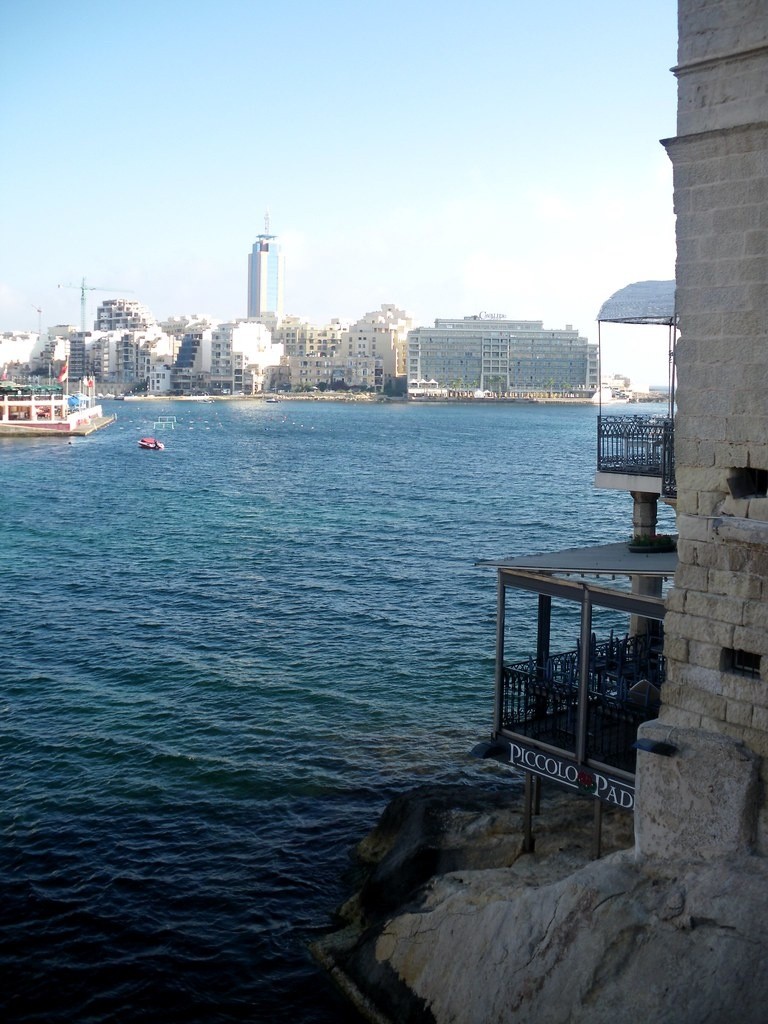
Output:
[727,463,768,499]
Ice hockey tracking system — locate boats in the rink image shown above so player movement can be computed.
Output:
[266,398,278,403]
[138,438,164,451]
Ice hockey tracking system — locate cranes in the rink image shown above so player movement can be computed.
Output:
[31,303,43,335]
[58,276,134,331]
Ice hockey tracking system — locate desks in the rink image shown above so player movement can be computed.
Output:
[525,656,664,769]
[637,423,663,465]
[650,644,664,655]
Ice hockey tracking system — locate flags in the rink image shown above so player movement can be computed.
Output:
[0,368,7,381]
[82,374,93,387]
[57,361,67,381]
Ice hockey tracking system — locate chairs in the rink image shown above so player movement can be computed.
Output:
[649,440,665,468]
[622,414,651,467]
[529,618,665,720]
